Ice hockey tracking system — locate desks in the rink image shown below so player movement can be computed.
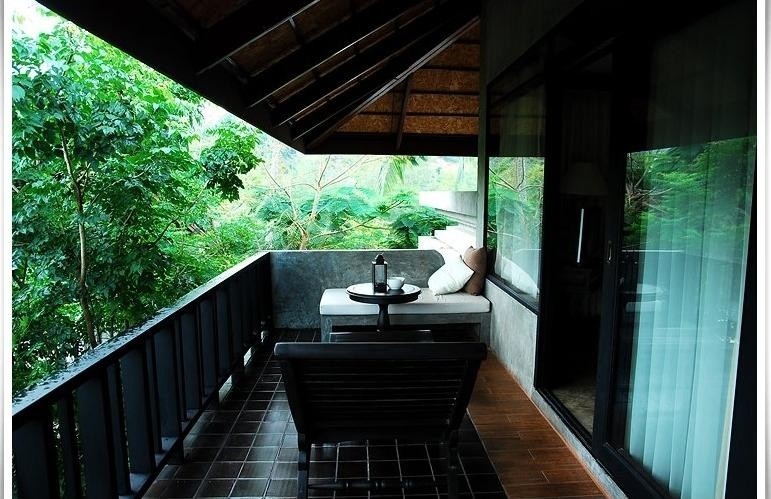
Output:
[345,279,422,329]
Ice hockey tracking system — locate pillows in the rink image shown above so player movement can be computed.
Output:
[427,243,490,298]
[486,247,540,300]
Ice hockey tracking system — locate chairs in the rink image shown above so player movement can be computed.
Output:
[270,340,487,498]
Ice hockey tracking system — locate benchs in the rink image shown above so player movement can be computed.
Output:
[317,284,493,343]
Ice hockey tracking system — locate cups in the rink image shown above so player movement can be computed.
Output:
[387,276,405,290]
